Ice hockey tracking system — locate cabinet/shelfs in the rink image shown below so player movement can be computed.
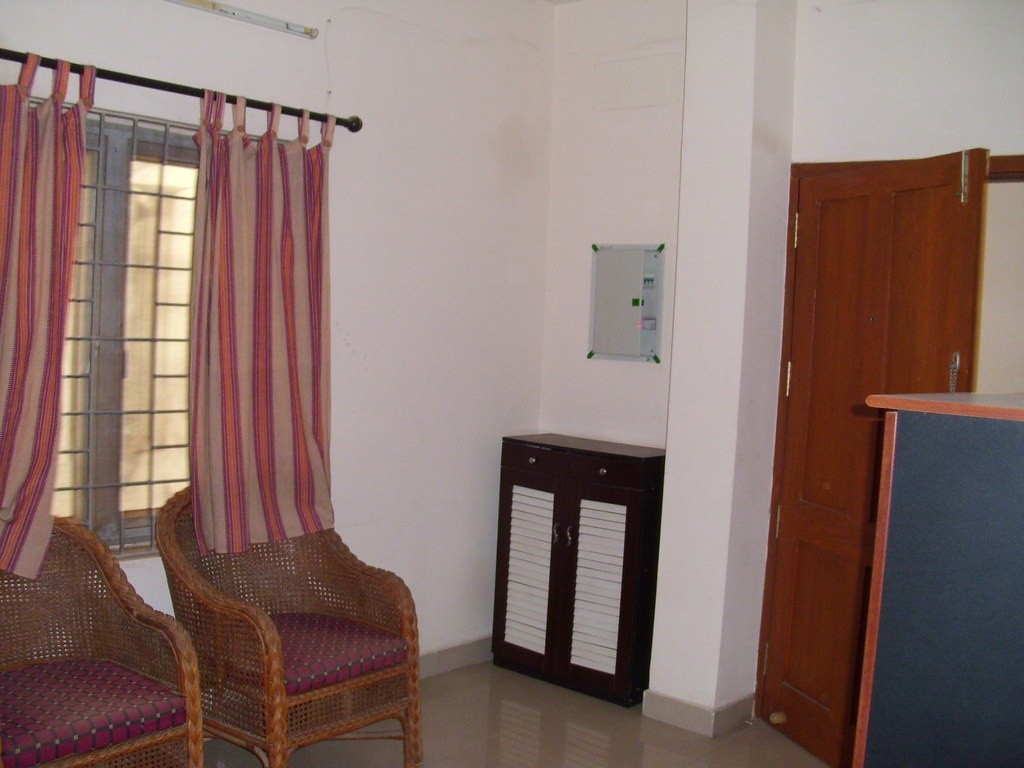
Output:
[492,434,666,708]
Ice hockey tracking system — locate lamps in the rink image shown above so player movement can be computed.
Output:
[168,0,319,38]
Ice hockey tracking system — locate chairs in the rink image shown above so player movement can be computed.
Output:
[0,515,205,768]
[156,487,423,768]
